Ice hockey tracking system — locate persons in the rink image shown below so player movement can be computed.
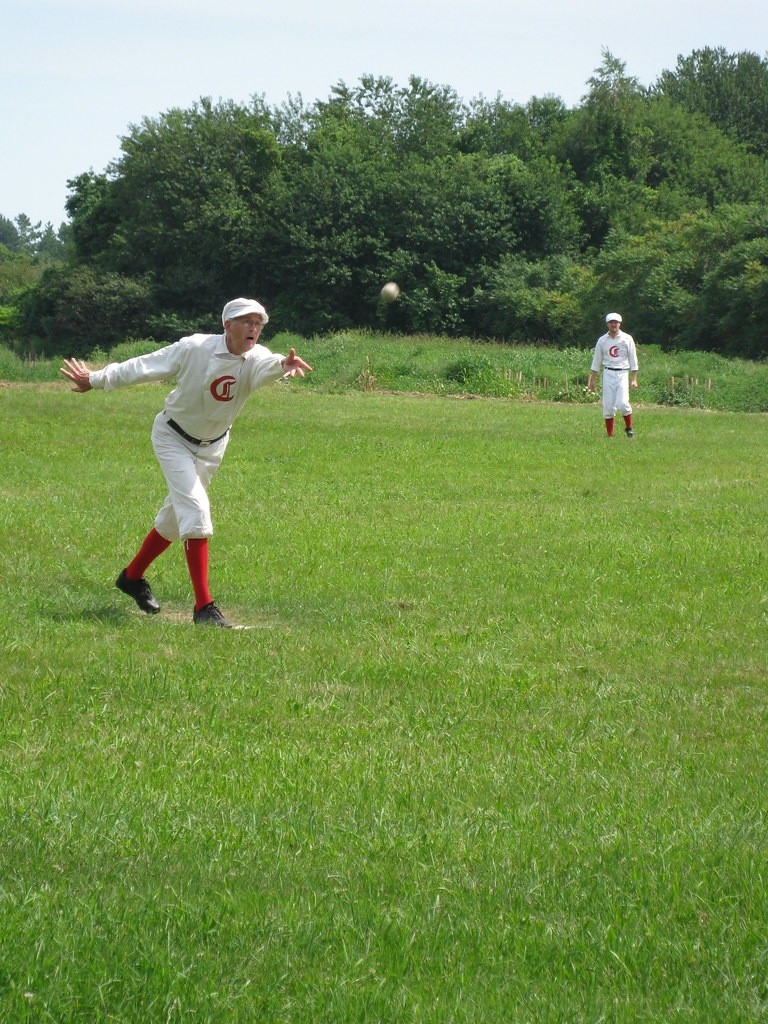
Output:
[59,298,313,629]
[589,313,639,438]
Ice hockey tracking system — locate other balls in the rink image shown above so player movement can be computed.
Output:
[381,283,401,304]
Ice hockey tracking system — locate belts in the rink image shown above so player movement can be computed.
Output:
[163,411,232,446]
[604,365,629,371]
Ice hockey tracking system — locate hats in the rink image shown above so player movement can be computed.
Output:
[605,312,622,323]
[221,296,269,324]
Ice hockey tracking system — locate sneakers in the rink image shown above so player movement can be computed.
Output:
[191,601,231,629]
[626,427,634,436]
[116,568,160,614]
